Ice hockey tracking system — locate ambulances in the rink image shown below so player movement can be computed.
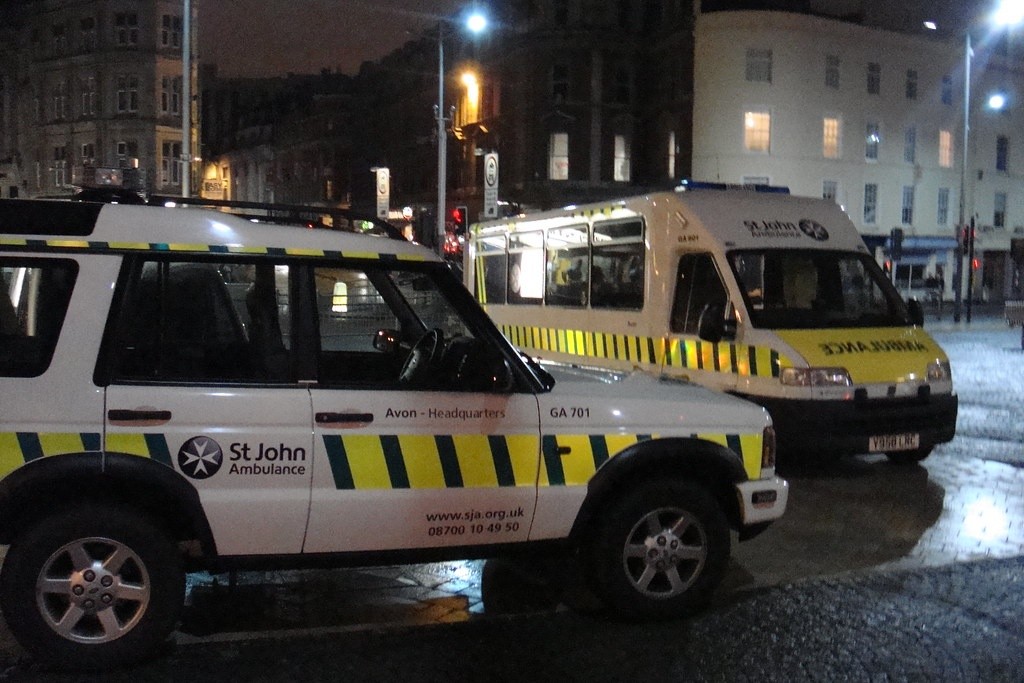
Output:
[0,182,789,683]
[466,180,960,463]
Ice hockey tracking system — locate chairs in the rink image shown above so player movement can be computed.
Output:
[36,261,286,382]
[780,257,818,309]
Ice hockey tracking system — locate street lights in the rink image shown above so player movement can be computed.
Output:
[433,7,493,258]
[953,0,1024,323]
